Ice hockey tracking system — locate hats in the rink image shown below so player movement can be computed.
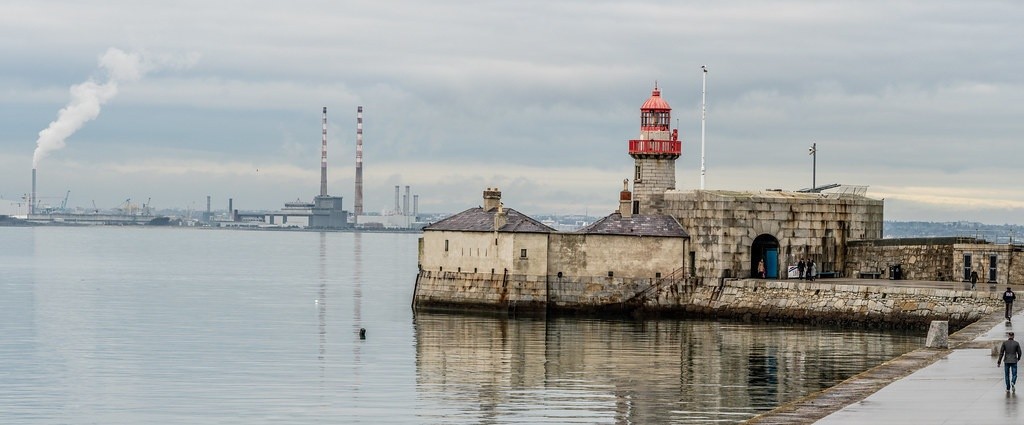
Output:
[1007,287,1011,289]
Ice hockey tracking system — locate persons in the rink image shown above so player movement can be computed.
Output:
[758,258,765,279]
[969,269,979,290]
[1003,286,1016,321]
[997,331,1022,392]
[798,258,816,281]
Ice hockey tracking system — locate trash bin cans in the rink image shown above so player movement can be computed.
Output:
[890,264,900,280]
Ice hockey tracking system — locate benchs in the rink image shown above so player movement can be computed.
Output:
[817,271,840,278]
[858,271,881,279]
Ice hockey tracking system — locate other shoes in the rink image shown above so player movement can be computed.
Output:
[1006,389,1010,392]
[1006,316,1011,321]
[1011,384,1015,392]
